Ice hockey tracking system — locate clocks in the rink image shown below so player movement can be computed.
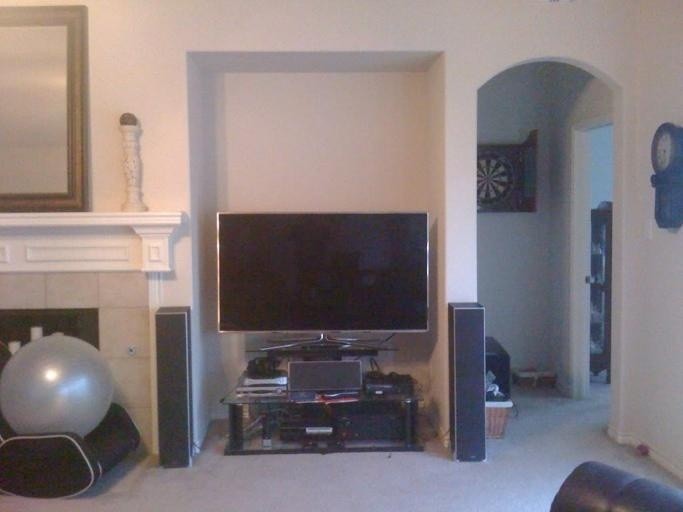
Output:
[649,121,683,230]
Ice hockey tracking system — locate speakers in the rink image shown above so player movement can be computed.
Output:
[155,305,194,468]
[448,302,486,463]
[287,360,363,391]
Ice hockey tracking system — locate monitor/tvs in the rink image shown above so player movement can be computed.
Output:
[216,211,429,351]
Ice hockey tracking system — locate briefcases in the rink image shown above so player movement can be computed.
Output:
[513,376,557,389]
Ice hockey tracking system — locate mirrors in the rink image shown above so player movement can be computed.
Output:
[0,5,86,211]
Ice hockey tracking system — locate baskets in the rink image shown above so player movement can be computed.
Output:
[486,407,511,439]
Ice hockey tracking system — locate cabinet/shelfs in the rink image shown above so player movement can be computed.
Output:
[590,201,612,383]
[220,380,424,456]
